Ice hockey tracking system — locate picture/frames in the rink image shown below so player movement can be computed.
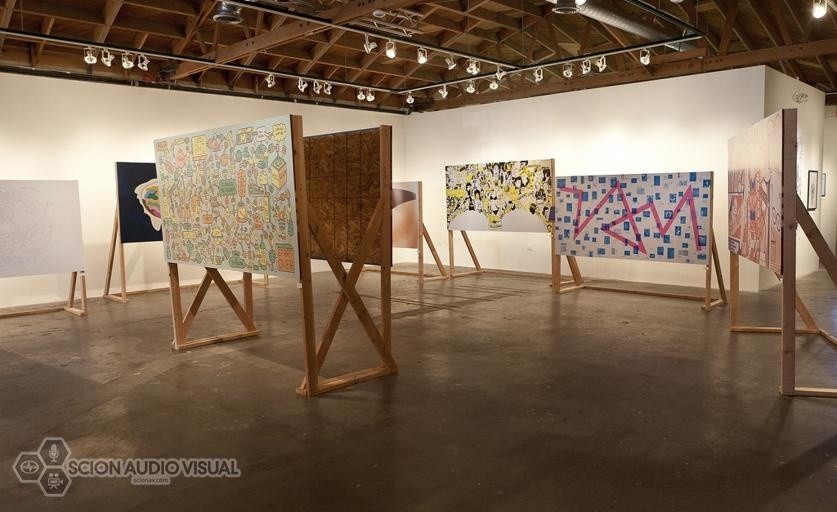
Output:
[807,170,818,210]
[821,173,826,197]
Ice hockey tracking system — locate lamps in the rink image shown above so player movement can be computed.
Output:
[812,0,828,19]
[85,47,150,71]
